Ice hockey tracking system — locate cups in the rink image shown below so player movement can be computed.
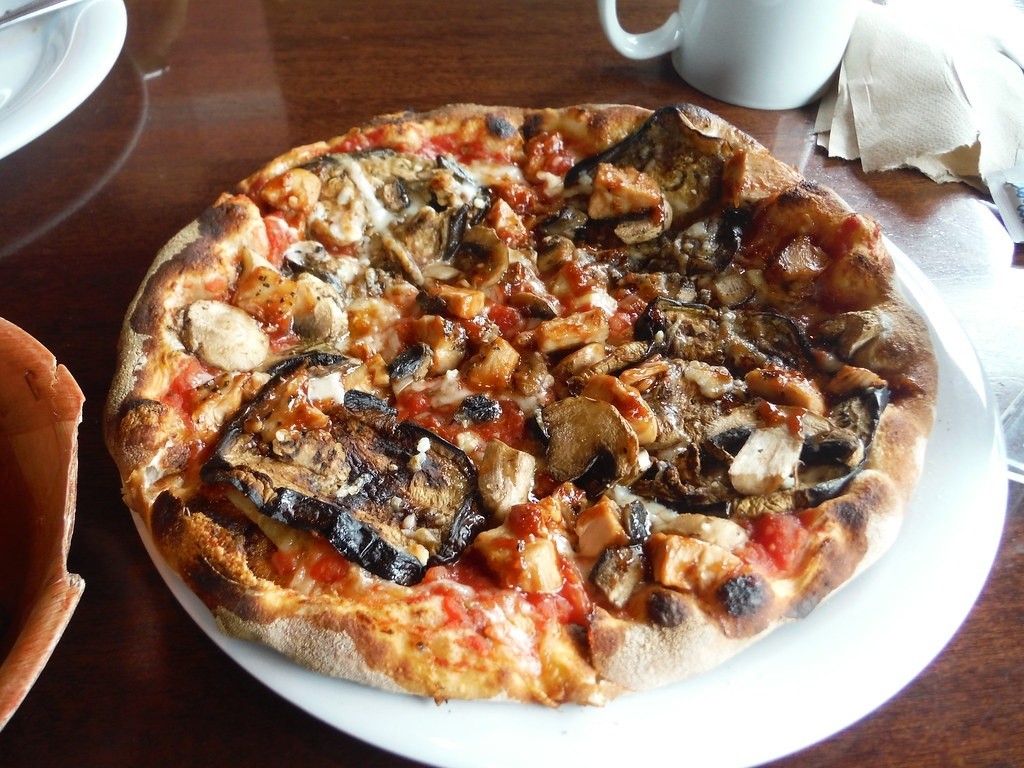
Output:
[596,1,868,109]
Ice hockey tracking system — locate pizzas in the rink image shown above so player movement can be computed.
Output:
[104,102,937,707]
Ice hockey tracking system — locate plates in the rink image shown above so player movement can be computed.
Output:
[1,36,166,266]
[109,105,1011,768]
[3,0,129,166]
